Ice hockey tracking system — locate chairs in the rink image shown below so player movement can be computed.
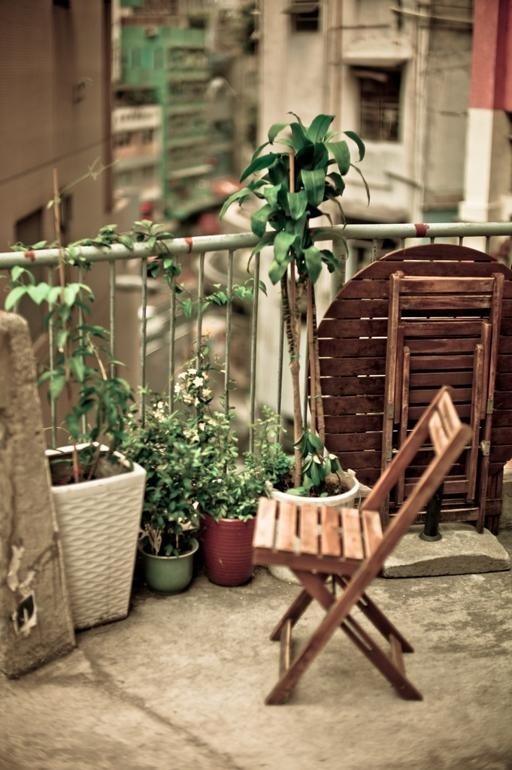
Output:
[253,386,474,705]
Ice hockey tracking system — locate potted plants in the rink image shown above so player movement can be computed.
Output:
[219,110,370,585]
[173,333,269,522]
[115,345,196,553]
[3,223,147,629]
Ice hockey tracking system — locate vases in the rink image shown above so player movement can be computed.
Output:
[136,534,199,594]
[200,512,257,585]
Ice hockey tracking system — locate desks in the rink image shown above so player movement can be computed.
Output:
[306,243,512,535]
